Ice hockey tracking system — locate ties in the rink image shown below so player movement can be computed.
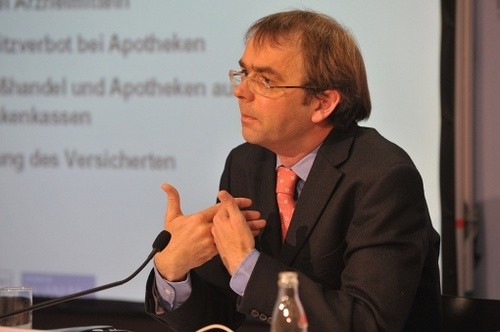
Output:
[276,167,297,244]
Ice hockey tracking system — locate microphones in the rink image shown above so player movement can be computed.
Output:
[0,230,172,323]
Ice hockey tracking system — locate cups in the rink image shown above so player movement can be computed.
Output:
[0,286,33,332]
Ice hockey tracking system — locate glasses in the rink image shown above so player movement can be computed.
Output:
[229,70,325,95]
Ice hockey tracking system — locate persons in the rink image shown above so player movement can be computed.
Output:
[144,10,442,331]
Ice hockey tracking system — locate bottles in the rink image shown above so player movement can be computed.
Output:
[269,271,309,332]
